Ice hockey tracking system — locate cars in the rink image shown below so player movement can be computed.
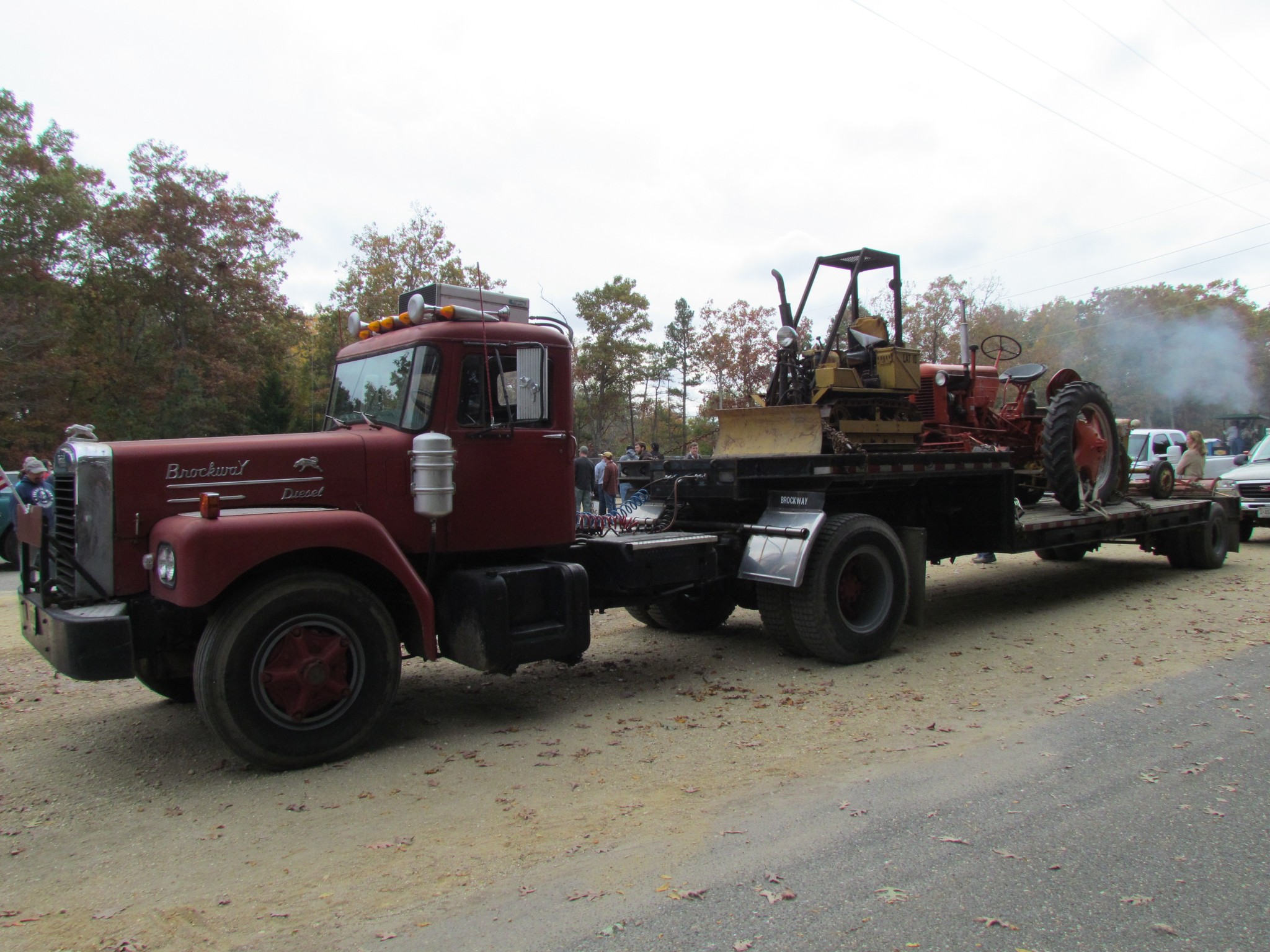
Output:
[0,469,54,567]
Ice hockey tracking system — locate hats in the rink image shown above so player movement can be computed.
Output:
[578,446,588,452]
[26,460,48,474]
[23,457,36,469]
[603,451,612,458]
[626,445,634,450]
[598,450,608,456]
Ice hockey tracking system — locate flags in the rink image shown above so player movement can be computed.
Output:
[0,466,12,491]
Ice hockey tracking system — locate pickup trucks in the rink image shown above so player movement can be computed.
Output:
[1126,428,1238,483]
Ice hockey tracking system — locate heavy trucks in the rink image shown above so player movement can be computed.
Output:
[14,280,1240,774]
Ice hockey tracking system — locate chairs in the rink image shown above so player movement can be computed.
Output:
[844,315,887,365]
[998,363,1047,383]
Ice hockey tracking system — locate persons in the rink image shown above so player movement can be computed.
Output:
[1175,431,1207,481]
[576,441,713,529]
[10,457,56,581]
[1222,420,1259,455]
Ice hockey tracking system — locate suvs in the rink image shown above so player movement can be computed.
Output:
[1209,428,1270,542]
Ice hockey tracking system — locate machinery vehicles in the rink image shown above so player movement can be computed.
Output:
[756,248,1122,512]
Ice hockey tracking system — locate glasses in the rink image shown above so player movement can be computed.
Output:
[651,442,654,447]
